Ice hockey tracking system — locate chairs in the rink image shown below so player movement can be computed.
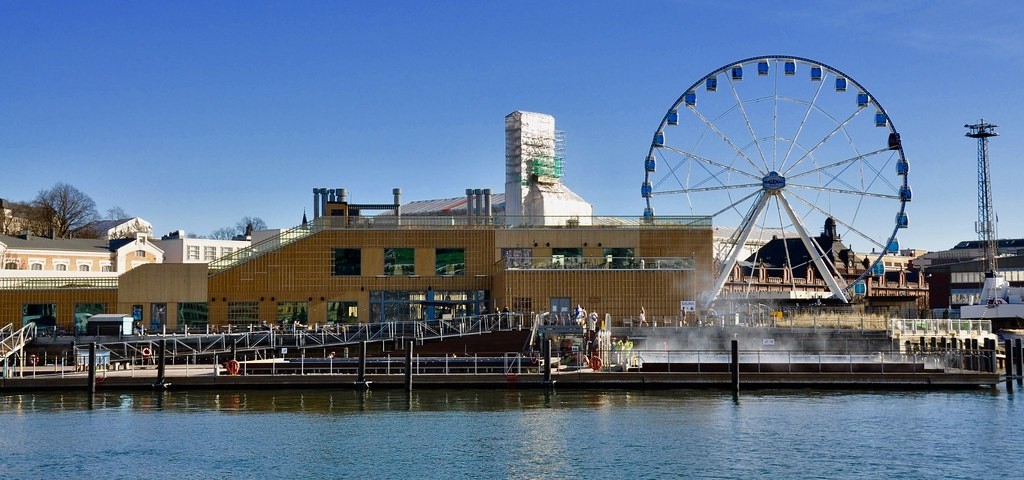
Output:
[623,318,634,327]
[664,317,671,326]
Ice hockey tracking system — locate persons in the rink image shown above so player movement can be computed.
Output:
[696,306,723,326]
[609,335,633,364]
[136,321,147,336]
[573,303,598,332]
[479,304,511,329]
[681,306,689,327]
[639,306,649,327]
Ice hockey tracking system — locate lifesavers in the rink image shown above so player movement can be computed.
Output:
[589,356,602,369]
[226,360,239,374]
[143,348,151,355]
[29,355,39,365]
